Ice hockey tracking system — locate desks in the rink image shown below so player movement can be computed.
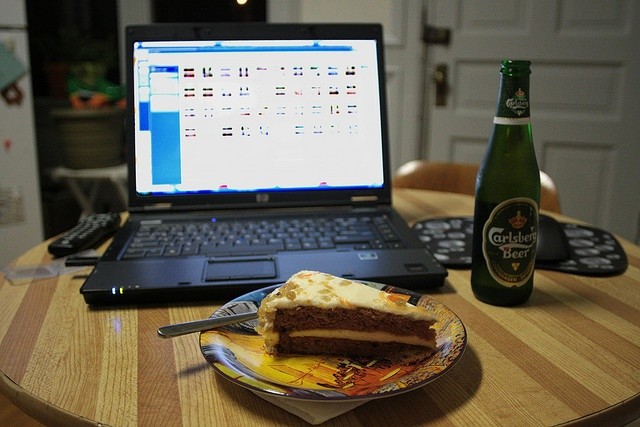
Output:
[54,164,128,221]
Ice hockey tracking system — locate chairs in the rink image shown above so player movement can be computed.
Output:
[395,161,558,209]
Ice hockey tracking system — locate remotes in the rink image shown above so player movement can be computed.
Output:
[47,210,120,259]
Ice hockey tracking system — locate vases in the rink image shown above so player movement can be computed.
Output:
[51,109,126,168]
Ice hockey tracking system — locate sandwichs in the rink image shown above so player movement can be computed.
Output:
[255,270,438,357]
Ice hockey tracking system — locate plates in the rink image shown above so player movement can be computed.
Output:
[200,276,466,403]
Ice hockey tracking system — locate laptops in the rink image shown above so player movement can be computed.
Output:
[78,21,449,309]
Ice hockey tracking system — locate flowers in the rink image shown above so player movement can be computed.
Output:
[67,55,126,109]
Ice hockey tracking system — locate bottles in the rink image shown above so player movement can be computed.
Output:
[471,59,541,307]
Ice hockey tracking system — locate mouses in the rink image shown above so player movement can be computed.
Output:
[533,212,574,266]
